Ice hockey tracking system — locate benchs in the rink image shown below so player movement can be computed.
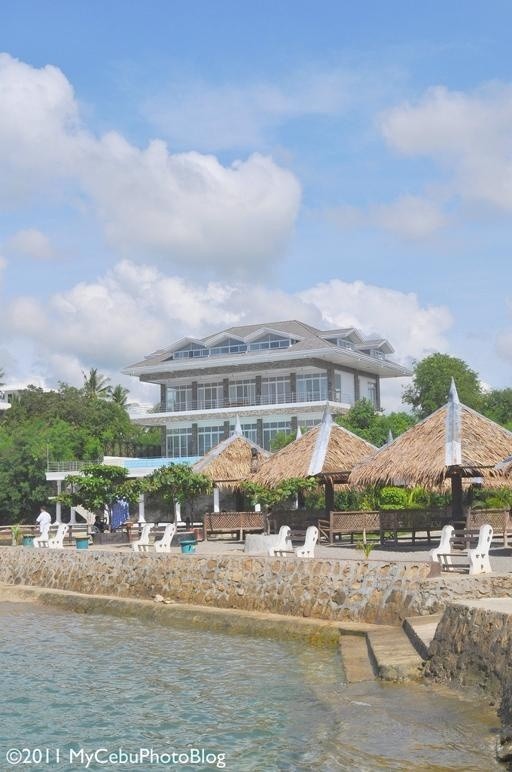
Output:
[32,522,68,548]
[448,510,509,549]
[379,508,432,545]
[203,511,270,542]
[131,523,176,553]
[269,525,317,559]
[426,524,494,576]
[318,510,380,545]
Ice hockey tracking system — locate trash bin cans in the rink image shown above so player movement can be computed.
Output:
[23,535,35,545]
[75,537,90,549]
[180,540,197,553]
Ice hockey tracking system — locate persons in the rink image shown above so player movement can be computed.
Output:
[34,506,52,540]
[94,515,109,534]
